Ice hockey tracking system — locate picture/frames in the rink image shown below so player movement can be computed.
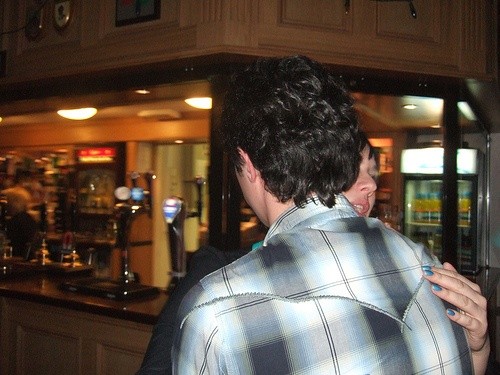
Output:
[115,0,162,27]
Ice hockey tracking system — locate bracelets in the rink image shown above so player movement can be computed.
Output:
[470,334,487,351]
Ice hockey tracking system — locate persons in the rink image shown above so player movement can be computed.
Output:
[172,56,476,375]
[0,170,47,258]
[136,131,490,375]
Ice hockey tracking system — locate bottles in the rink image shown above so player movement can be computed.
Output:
[411,191,469,226]
[456,229,473,274]
[409,229,441,260]
